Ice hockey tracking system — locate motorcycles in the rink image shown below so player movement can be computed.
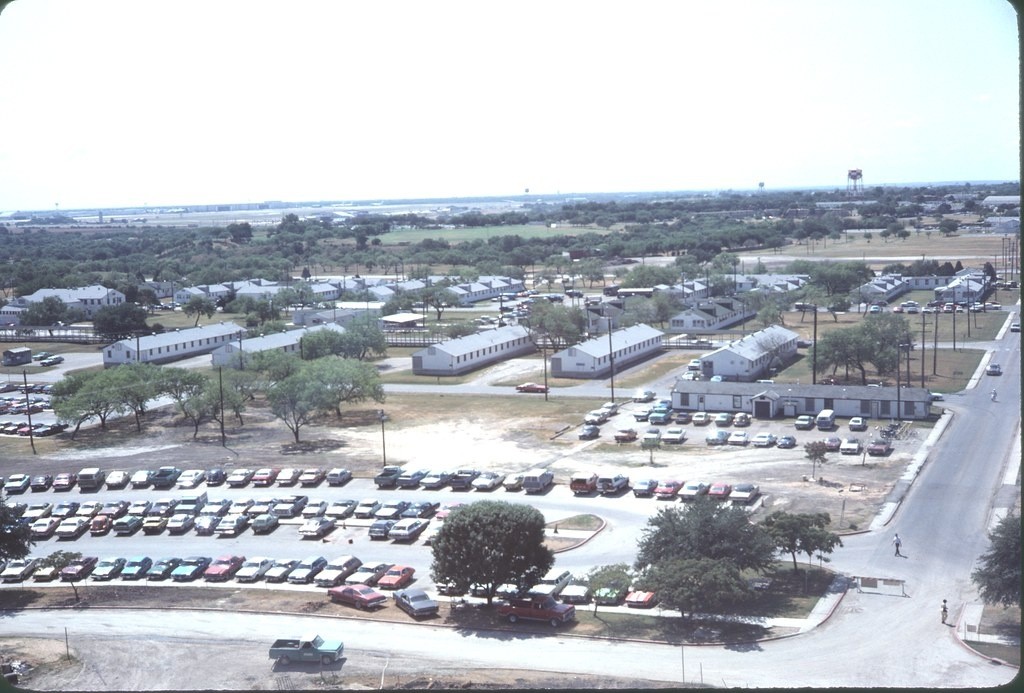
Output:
[991,395,997,401]
[879,423,905,440]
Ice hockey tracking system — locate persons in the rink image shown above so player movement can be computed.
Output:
[940,599,948,624]
[991,389,997,400]
[892,533,902,556]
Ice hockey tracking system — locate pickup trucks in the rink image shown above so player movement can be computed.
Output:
[269,635,344,666]
[273,495,309,517]
[650,407,673,425]
[373,466,406,487]
[150,466,181,488]
[448,470,482,490]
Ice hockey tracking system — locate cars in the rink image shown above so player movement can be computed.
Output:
[516,383,549,393]
[986,363,1001,375]
[929,390,943,401]
[0,470,760,627]
[471,286,590,325]
[578,358,892,456]
[1010,323,1020,333]
[870,301,1002,313]
[1,351,69,437]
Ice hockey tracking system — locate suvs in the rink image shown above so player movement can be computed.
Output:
[77,467,105,490]
[520,469,554,492]
[569,471,598,495]
[173,490,208,515]
[596,474,629,495]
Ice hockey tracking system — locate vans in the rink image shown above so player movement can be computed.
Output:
[817,410,835,430]
